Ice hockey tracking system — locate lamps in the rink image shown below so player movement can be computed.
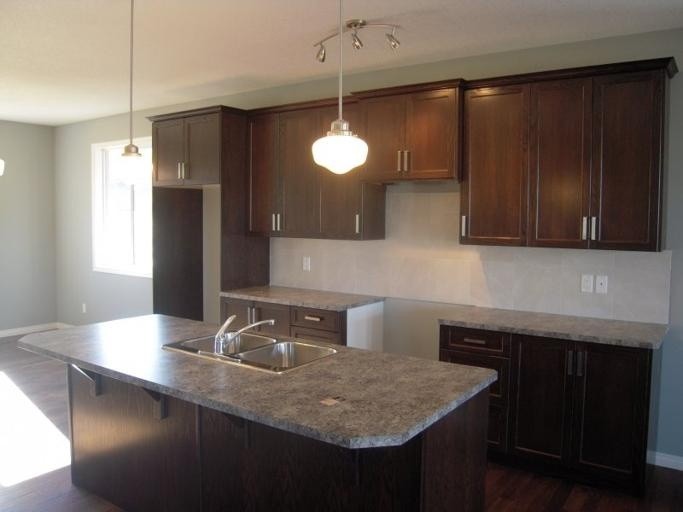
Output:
[107,1,155,186]
[309,1,405,177]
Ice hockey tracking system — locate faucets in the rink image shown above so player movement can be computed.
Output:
[214,314,277,354]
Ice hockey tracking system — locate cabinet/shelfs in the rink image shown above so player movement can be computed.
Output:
[247,110,318,236]
[528,71,662,253]
[460,83,528,246]
[219,285,386,353]
[150,113,219,188]
[322,105,386,242]
[359,89,455,183]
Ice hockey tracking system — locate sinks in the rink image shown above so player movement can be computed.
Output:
[223,341,340,374]
[160,328,279,357]
